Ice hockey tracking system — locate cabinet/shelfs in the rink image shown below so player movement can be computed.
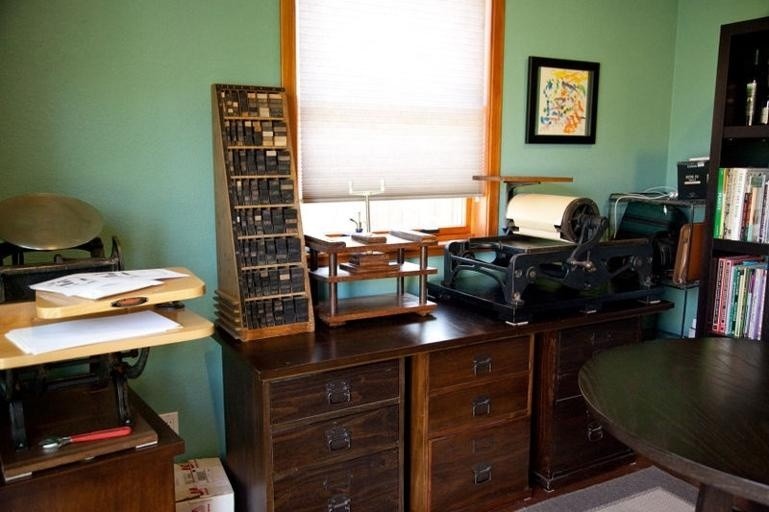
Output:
[697,16,768,340]
[307,228,440,325]
[210,81,319,340]
[216,299,677,512]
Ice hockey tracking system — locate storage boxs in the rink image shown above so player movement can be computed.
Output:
[173,457,235,512]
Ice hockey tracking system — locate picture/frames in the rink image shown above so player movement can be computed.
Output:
[524,55,601,145]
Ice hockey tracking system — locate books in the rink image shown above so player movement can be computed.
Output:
[706,167,769,341]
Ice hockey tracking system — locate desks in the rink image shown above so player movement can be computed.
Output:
[579,335,769,510]
